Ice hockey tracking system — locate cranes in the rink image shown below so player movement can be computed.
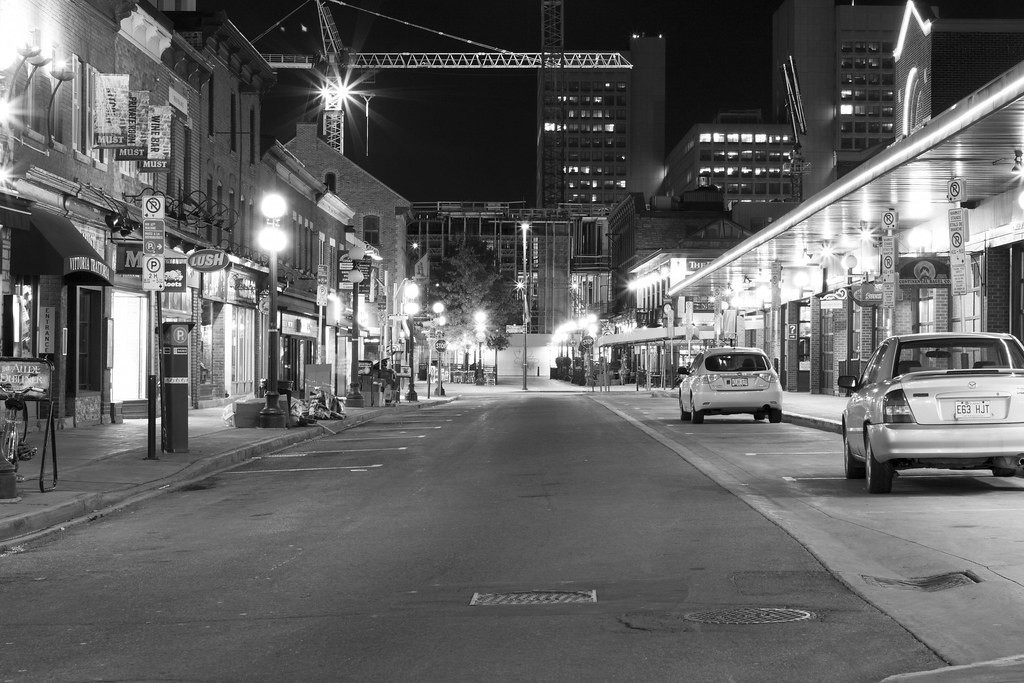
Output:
[249,0,635,156]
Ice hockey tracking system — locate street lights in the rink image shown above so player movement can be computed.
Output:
[432,302,447,396]
[474,311,485,385]
[254,191,287,429]
[404,283,421,401]
[520,221,529,390]
[345,245,365,407]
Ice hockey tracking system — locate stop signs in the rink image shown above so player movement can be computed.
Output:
[581,335,595,349]
[433,339,448,353]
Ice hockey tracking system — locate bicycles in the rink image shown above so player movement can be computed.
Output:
[0,381,46,462]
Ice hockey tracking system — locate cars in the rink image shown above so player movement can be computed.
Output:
[838,331,1024,494]
[676,347,784,424]
